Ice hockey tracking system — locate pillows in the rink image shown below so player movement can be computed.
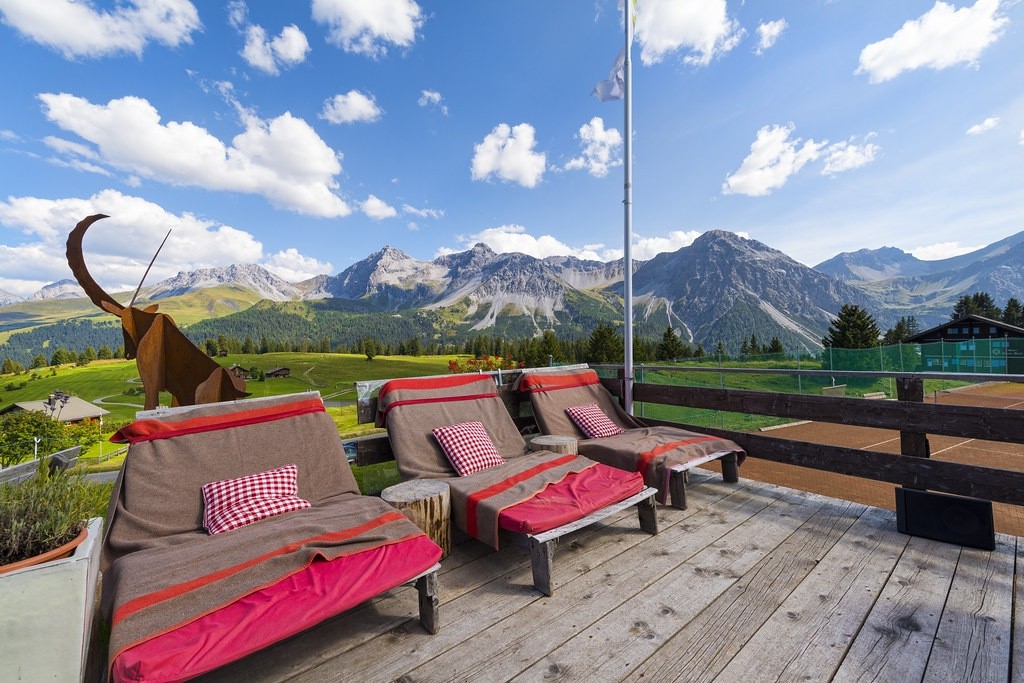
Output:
[431,420,506,478]
[202,464,310,534]
[565,402,625,440]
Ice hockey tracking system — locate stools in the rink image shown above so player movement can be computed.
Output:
[379,479,451,560]
[530,435,578,458]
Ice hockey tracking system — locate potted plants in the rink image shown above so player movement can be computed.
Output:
[0,447,88,574]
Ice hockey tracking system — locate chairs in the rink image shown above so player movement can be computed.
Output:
[113,389,442,682]
[515,366,746,510]
[375,374,658,595]
